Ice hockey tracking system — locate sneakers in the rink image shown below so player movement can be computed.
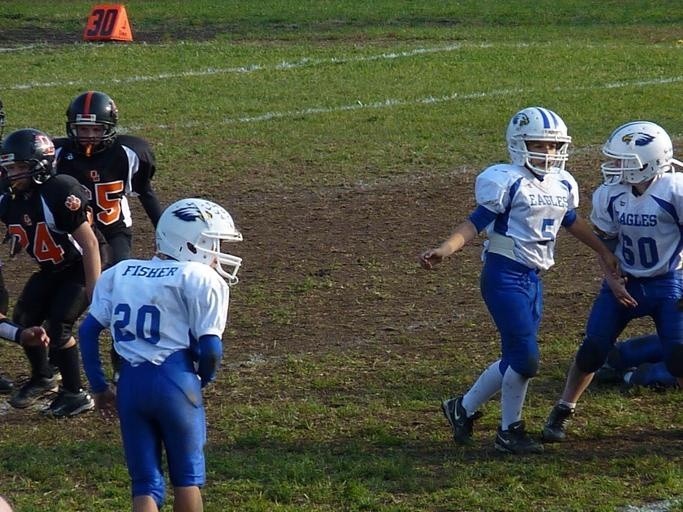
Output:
[594,366,633,385]
[0,368,14,394]
[39,384,97,423]
[541,403,579,443]
[494,419,546,457]
[441,394,485,449]
[8,372,60,410]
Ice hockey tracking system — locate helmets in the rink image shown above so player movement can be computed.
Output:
[65,90,119,139]
[604,118,674,185]
[0,127,57,185]
[505,105,568,168]
[154,195,240,268]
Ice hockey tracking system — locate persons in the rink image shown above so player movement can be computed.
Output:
[76,195,240,512]
[49,90,164,274]
[0,129,110,418]
[543,119,681,442]
[0,100,14,392]
[601,332,675,386]
[418,105,624,455]
[0,313,51,350]
